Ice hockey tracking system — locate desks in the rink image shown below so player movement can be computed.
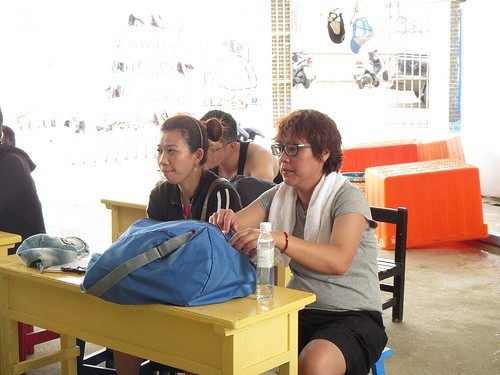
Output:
[0,250,316,375]
[102,198,288,290]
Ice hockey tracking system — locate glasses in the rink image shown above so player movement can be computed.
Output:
[271,144,313,157]
[208,141,231,155]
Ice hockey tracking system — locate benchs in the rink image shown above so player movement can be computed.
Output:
[372,205,410,324]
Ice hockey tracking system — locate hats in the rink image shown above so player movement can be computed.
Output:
[16,233,95,272]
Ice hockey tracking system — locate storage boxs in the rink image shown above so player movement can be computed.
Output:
[364,157,491,249]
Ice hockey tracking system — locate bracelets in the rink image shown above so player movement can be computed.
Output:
[280,231,289,254]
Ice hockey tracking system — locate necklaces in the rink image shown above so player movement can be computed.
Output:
[180,192,195,220]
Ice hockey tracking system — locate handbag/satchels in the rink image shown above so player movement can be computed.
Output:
[80,218,258,307]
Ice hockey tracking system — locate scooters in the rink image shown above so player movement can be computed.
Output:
[351,48,382,89]
[291,51,317,89]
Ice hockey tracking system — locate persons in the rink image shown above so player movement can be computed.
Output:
[113,115,243,375]
[0,110,47,254]
[200,110,283,185]
[209,109,388,375]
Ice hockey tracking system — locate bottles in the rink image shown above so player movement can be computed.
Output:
[256,223,274,301]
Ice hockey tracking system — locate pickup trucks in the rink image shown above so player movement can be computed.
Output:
[389,53,428,103]
